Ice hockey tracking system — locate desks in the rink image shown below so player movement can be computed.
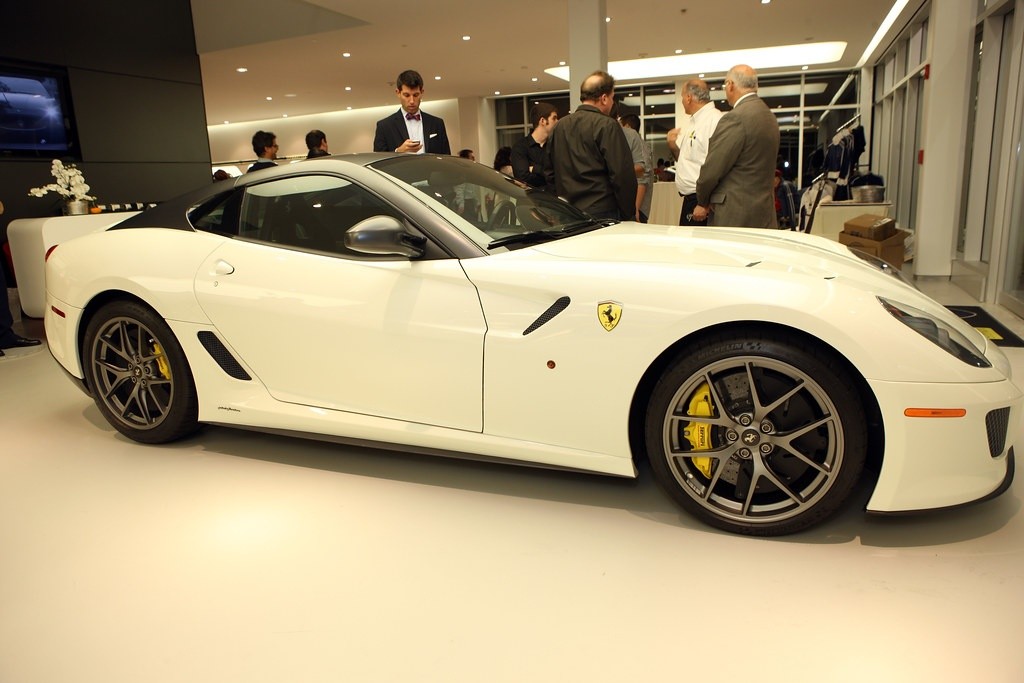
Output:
[7,211,142,318]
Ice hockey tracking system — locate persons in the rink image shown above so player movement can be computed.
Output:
[452,149,480,224]
[300,129,348,210]
[773,168,785,224]
[491,146,521,228]
[543,70,674,224]
[213,130,279,230]
[667,79,724,226]
[372,70,457,212]
[692,65,781,229]
[0,203,42,357]
[510,103,560,224]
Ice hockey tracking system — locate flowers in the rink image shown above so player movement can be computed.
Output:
[26,159,98,201]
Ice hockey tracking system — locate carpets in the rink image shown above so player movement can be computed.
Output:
[945,305,1024,348]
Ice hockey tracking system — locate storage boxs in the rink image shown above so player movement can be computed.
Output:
[839,214,911,269]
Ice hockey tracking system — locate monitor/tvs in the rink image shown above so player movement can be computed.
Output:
[0,56,83,162]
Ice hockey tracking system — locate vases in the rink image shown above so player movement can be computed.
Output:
[64,200,89,215]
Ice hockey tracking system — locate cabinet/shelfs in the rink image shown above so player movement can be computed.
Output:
[816,204,892,244]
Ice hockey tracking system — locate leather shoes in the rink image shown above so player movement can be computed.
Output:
[0,350,5,356]
[0,335,41,349]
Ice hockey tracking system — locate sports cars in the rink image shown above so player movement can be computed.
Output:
[45,153,1016,537]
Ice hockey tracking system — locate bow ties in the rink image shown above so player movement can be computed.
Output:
[405,113,420,121]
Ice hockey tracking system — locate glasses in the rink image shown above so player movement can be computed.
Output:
[272,144,279,150]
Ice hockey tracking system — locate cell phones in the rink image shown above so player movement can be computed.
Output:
[411,140,420,146]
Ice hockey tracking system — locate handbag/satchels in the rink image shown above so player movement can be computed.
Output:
[528,129,558,198]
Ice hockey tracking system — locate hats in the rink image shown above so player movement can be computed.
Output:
[774,170,783,177]
